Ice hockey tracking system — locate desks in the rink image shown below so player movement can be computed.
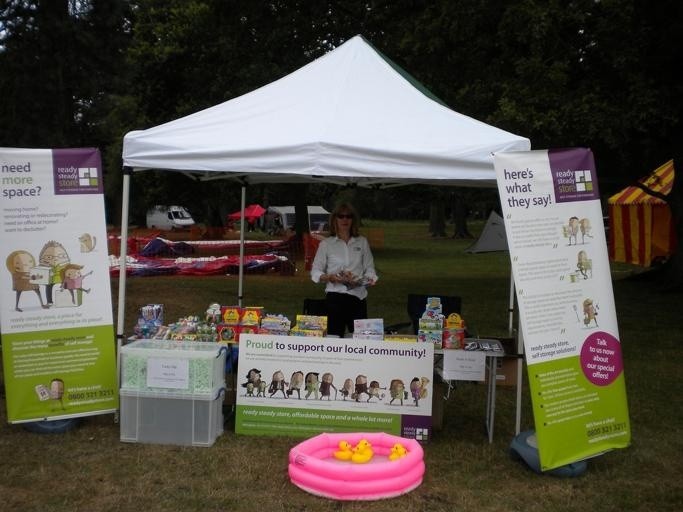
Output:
[386,332,506,446]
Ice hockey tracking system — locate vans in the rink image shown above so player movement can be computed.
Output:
[145,204,196,232]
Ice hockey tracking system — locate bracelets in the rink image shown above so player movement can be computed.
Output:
[329,275,335,283]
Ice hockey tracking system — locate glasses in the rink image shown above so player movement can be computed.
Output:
[41,252,68,261]
[335,213,353,220]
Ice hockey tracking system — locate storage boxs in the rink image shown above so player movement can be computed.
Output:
[434,383,445,430]
[117,388,225,448]
[120,339,229,394]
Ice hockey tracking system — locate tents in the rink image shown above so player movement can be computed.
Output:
[606,157,677,268]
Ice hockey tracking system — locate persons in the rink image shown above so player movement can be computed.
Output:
[310,201,379,338]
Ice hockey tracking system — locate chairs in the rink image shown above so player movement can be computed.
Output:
[302,298,328,314]
[383,293,464,402]
